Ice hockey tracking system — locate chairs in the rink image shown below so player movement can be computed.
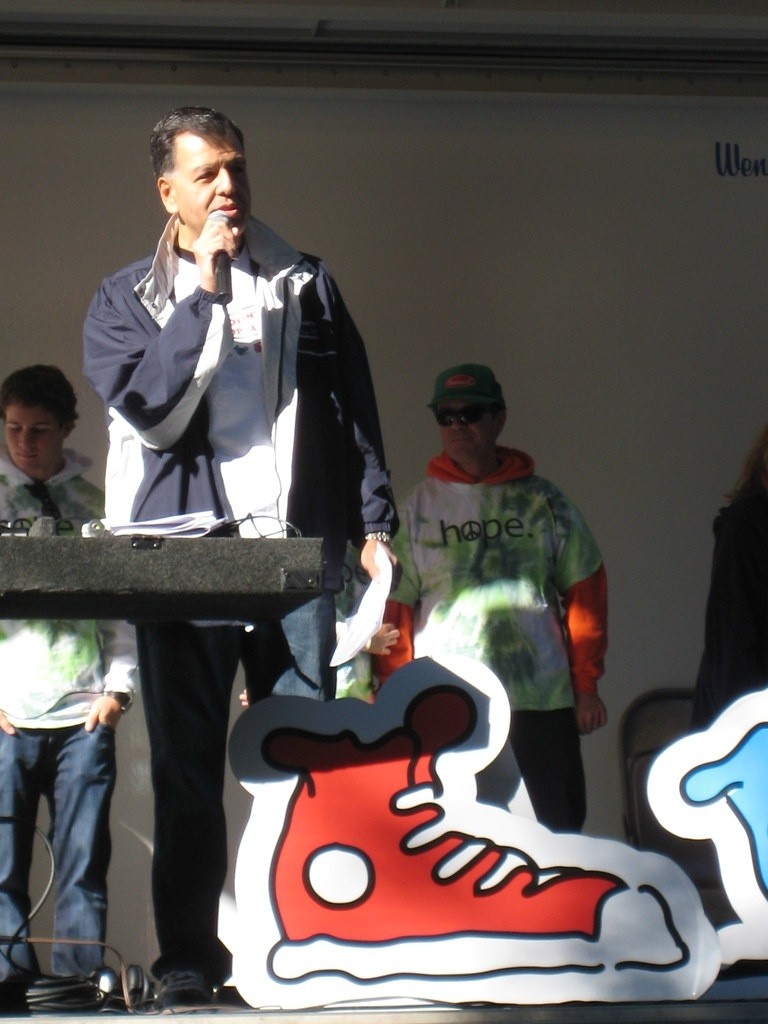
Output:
[618,686,722,889]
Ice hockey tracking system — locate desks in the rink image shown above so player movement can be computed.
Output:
[0,535,329,625]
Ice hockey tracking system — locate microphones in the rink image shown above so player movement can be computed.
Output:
[209,209,234,304]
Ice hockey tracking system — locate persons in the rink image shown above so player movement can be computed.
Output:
[380,365,611,835]
[691,413,768,729]
[81,106,397,1005]
[0,365,140,994]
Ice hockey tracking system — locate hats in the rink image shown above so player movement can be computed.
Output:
[428,363,505,409]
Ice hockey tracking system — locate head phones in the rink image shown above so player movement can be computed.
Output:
[32,965,153,1005]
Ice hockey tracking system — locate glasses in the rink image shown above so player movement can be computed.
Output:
[24,483,63,522]
[433,406,489,428]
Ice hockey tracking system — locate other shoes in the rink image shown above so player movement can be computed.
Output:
[160,971,213,1004]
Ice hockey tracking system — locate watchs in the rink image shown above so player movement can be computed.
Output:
[105,689,133,713]
[363,531,391,543]
